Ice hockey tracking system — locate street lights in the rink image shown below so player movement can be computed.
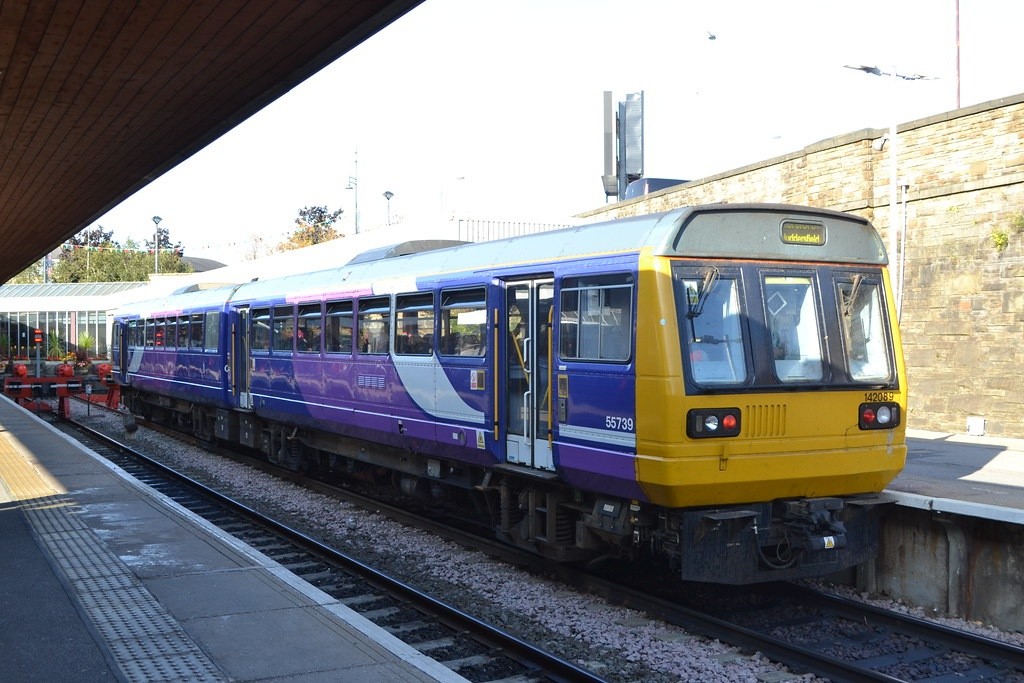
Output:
[843,65,941,329]
[152,216,163,274]
[383,191,394,224]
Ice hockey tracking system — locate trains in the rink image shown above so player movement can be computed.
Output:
[111,188,908,615]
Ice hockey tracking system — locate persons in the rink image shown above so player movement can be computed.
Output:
[276,319,481,358]
[127,325,202,348]
[766,285,798,359]
[4,358,16,375]
